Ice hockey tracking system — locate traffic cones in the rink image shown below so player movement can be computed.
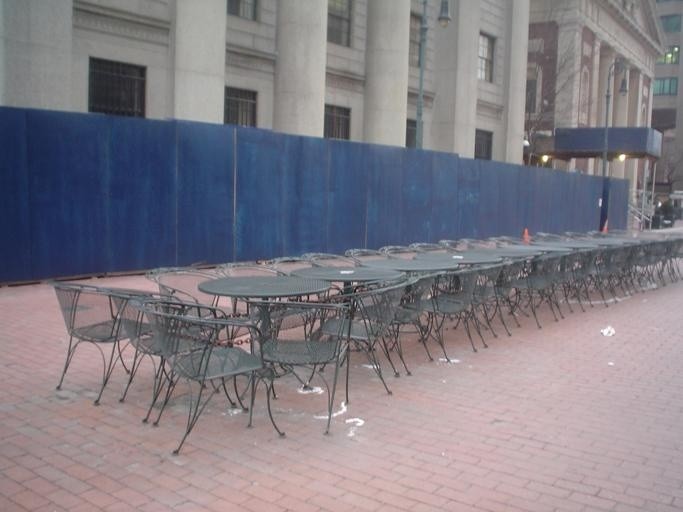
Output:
[522,227,530,246]
[601,218,609,234]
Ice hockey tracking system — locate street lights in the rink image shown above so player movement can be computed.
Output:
[415,0,454,149]
[600,57,629,231]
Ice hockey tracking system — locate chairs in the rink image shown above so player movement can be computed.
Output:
[53,227,683,458]
